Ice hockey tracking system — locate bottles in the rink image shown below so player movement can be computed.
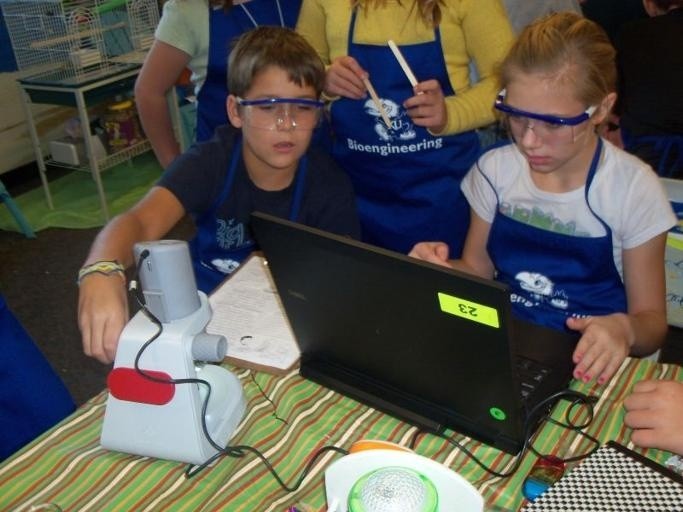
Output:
[104,100,139,146]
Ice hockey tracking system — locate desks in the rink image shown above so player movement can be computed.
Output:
[1,352,683,512]
[16,62,188,226]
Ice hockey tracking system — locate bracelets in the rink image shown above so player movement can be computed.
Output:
[76,259,126,283]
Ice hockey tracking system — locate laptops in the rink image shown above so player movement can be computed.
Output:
[250,210,582,456]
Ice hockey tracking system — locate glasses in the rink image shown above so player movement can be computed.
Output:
[494,88,599,143]
[236,98,324,130]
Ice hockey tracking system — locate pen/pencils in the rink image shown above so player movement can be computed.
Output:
[387,38,422,97]
[360,74,394,130]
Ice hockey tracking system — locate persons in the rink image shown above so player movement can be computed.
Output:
[76,26,358,364]
[134,0,303,171]
[295,1,516,262]
[407,11,679,386]
[611,0,683,136]
[623,379,683,456]
